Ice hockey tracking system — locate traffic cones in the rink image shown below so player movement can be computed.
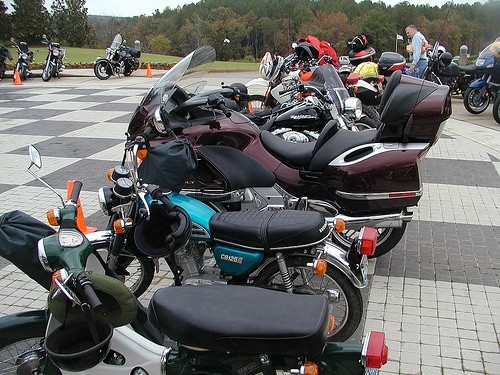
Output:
[146,64,152,77]
[13,69,23,85]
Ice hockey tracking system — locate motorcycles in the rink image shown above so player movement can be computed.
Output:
[41,34,67,82]
[0,43,13,79]
[10,36,39,82]
[1,36,500,375]
[93,33,142,80]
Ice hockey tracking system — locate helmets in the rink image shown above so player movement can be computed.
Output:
[439,52,453,67]
[295,42,319,60]
[46,315,114,373]
[438,46,446,53]
[133,199,192,259]
[258,52,284,81]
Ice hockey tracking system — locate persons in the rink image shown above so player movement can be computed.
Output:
[405,25,429,77]
